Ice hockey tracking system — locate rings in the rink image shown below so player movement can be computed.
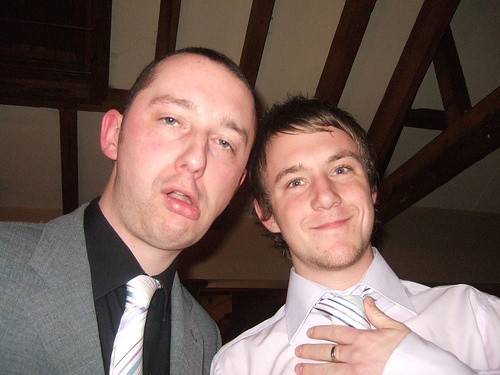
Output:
[330,345,339,362]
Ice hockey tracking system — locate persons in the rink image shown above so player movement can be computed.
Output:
[0,47,258,375]
[210,94,500,375]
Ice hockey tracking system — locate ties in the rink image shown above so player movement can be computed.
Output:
[314,285,374,329]
[109,274,163,375]
